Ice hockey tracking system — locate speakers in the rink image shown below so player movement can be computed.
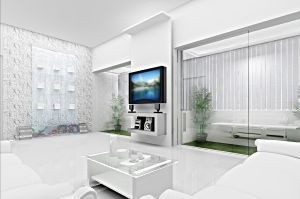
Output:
[154,104,164,113]
[128,105,136,113]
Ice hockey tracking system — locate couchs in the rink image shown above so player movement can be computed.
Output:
[1,140,97,199]
[160,138,300,199]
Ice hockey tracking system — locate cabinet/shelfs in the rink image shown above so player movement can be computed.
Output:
[128,113,166,136]
[212,122,300,140]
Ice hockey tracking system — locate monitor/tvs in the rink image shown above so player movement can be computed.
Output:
[129,66,166,113]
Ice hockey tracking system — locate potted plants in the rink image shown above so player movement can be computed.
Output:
[110,93,126,131]
[190,85,213,143]
